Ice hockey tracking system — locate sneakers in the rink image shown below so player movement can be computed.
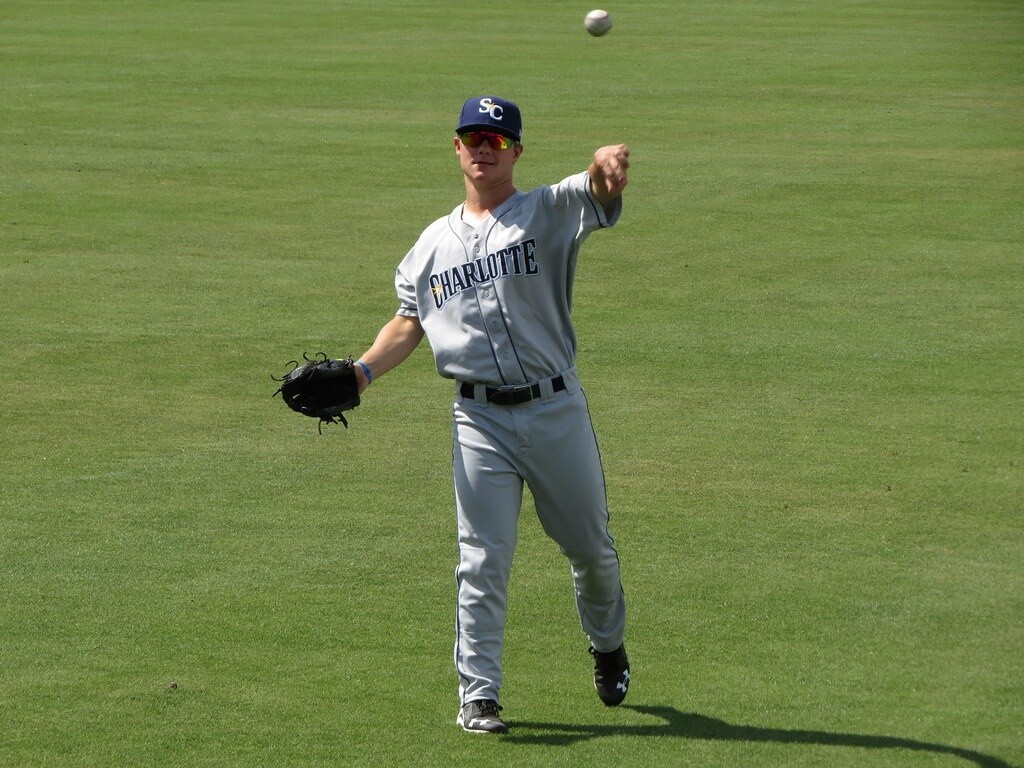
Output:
[456,699,508,734]
[587,642,630,707]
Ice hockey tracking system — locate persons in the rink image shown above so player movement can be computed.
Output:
[330,97,629,736]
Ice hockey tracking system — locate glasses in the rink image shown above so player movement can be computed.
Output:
[457,131,518,150]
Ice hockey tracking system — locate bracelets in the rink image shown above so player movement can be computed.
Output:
[352,360,373,386]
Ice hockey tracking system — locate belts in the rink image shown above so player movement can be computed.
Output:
[460,375,565,406]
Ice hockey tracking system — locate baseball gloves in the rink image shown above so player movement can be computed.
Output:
[277,358,362,418]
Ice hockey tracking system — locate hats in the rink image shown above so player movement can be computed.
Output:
[455,95,522,141]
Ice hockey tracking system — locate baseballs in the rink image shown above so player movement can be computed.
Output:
[584,8,613,38]
